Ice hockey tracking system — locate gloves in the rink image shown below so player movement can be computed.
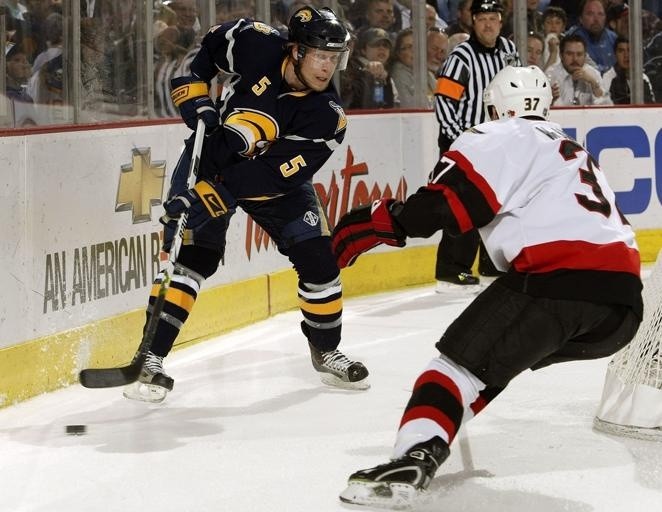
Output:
[331,198,402,268]
[170,77,218,134]
[159,175,240,232]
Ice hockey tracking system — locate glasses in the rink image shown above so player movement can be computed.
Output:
[428,26,449,35]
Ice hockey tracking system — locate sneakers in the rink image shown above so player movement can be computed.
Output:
[130,347,174,391]
[311,350,369,382]
[435,268,479,285]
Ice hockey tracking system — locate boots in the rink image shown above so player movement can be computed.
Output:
[349,435,450,492]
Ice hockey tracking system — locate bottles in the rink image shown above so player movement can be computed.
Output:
[373,79,384,108]
[545,68,556,87]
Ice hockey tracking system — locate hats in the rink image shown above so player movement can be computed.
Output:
[81,17,114,56]
[5,40,23,59]
[153,20,181,41]
[362,27,393,47]
[608,3,630,22]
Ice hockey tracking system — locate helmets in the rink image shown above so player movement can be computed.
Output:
[289,5,350,72]
[481,64,554,120]
[469,0,503,13]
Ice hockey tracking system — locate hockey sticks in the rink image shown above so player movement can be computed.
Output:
[80,120,206,389]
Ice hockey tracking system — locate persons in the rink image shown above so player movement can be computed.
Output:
[433,0,560,286]
[130,5,370,392]
[328,63,643,491]
